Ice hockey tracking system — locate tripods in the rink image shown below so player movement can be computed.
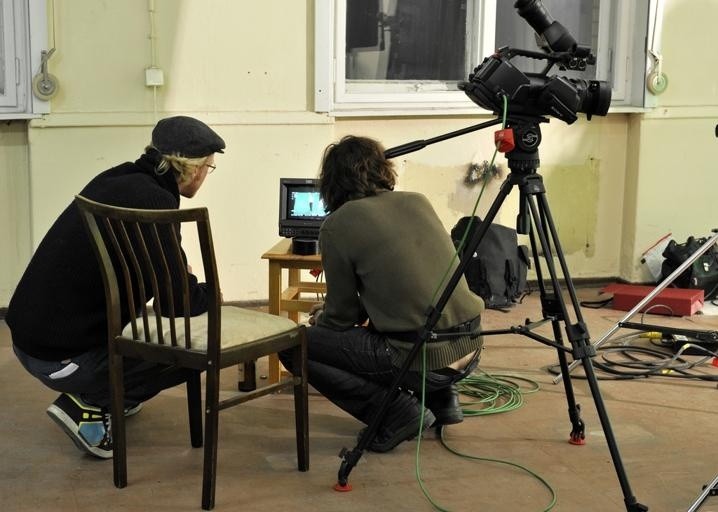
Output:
[334,120,648,512]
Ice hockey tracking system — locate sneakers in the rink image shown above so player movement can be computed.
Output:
[359,401,436,453]
[426,384,464,425]
[124,403,143,416]
[47,391,113,459]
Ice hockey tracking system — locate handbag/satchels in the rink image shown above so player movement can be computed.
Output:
[661,237,717,302]
[451,215,531,307]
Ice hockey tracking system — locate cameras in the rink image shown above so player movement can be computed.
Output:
[458,0,612,125]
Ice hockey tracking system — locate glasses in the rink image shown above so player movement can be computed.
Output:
[203,162,216,174]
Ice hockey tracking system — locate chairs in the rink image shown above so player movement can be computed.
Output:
[75,195,309,509]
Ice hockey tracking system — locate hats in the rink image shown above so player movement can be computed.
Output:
[152,115,226,158]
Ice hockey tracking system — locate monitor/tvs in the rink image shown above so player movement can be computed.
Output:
[279,178,331,255]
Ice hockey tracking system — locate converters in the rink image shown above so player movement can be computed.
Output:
[672,334,718,355]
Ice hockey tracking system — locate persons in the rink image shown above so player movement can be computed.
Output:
[276,131,485,454]
[0,115,226,459]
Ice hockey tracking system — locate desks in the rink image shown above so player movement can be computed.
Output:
[262,237,330,395]
